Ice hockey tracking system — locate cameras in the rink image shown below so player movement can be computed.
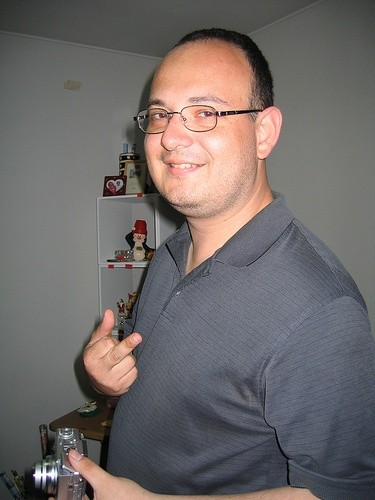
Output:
[31,428,89,500]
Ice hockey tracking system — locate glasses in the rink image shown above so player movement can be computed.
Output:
[133,105,264,134]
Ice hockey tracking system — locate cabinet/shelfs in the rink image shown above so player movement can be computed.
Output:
[95,186,181,337]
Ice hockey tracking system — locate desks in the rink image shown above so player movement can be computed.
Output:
[50,395,115,467]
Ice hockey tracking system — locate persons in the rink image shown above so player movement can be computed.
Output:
[49,28,375,500]
